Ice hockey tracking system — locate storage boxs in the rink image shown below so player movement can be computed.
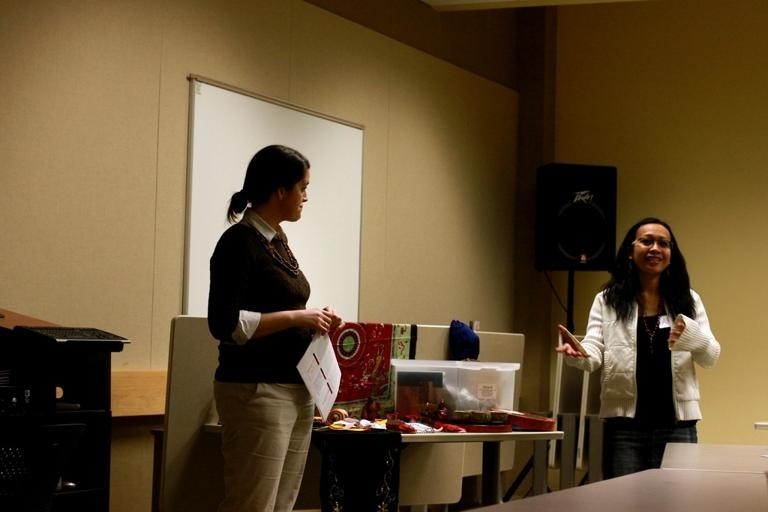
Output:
[390,358,519,418]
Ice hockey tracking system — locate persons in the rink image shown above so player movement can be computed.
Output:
[207,145,345,512]
[555,218,721,481]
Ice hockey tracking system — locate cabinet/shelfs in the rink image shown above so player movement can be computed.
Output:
[0,327,130,508]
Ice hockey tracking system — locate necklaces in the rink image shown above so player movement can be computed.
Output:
[639,301,664,355]
[244,219,299,277]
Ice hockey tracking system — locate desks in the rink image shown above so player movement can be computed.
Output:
[316,416,564,511]
[471,440,764,512]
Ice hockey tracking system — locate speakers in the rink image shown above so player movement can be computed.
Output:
[534,163,617,272]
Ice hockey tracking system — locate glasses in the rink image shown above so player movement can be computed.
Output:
[633,237,673,249]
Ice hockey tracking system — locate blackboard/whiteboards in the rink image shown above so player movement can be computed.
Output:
[182,75,364,324]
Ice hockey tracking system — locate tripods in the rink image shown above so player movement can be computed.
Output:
[500,274,592,503]
